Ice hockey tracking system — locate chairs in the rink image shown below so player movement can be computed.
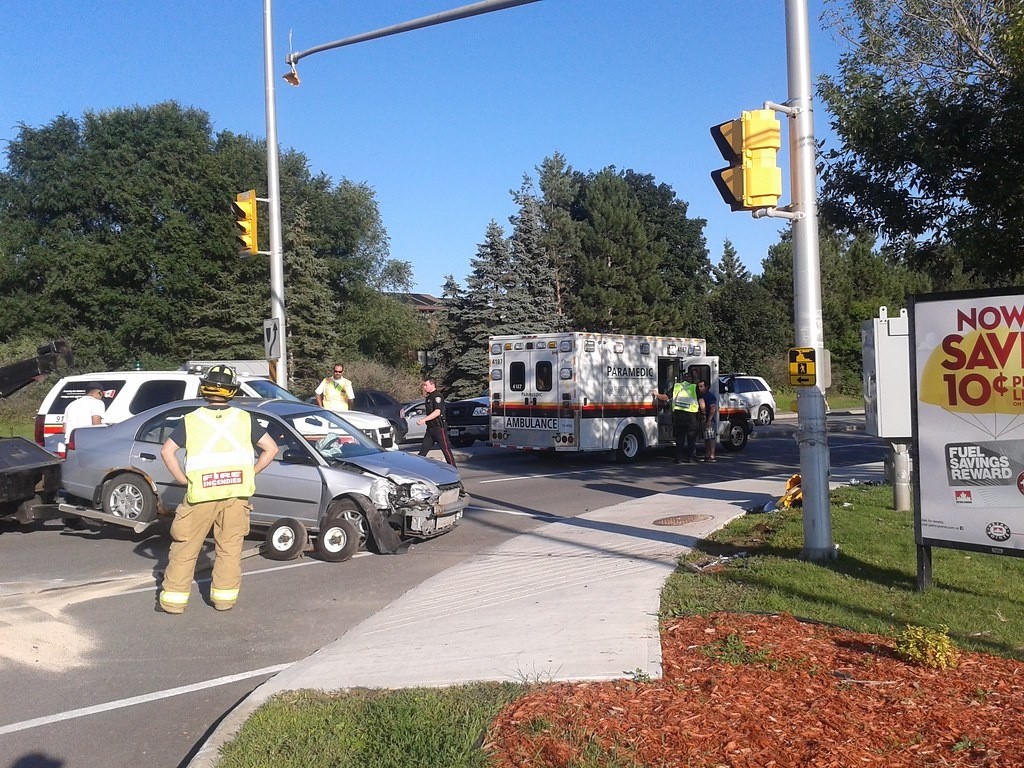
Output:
[265,418,299,459]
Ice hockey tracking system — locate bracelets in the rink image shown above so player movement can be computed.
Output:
[703,414,707,415]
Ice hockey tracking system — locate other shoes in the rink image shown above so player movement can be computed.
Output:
[700,455,717,462]
[683,455,697,463]
[672,456,681,463]
[64,516,90,532]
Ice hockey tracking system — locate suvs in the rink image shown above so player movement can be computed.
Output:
[446,385,498,448]
[716,372,777,425]
[33,367,401,494]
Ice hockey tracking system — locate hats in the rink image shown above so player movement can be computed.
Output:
[87,382,104,392]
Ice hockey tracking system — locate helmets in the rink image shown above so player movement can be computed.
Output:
[199,364,241,390]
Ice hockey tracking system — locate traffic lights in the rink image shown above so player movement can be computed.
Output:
[710,107,782,207]
[230,189,258,256]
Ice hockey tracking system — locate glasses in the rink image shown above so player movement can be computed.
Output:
[335,371,343,373]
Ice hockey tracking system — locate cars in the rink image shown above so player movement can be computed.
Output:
[61,393,473,551]
[400,400,451,443]
[305,388,411,448]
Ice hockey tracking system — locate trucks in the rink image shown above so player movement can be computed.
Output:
[488,331,754,464]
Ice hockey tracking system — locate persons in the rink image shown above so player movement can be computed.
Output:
[315,363,355,411]
[61,381,109,532]
[650,373,707,464]
[417,377,457,471]
[696,380,718,462]
[156,363,278,613]
